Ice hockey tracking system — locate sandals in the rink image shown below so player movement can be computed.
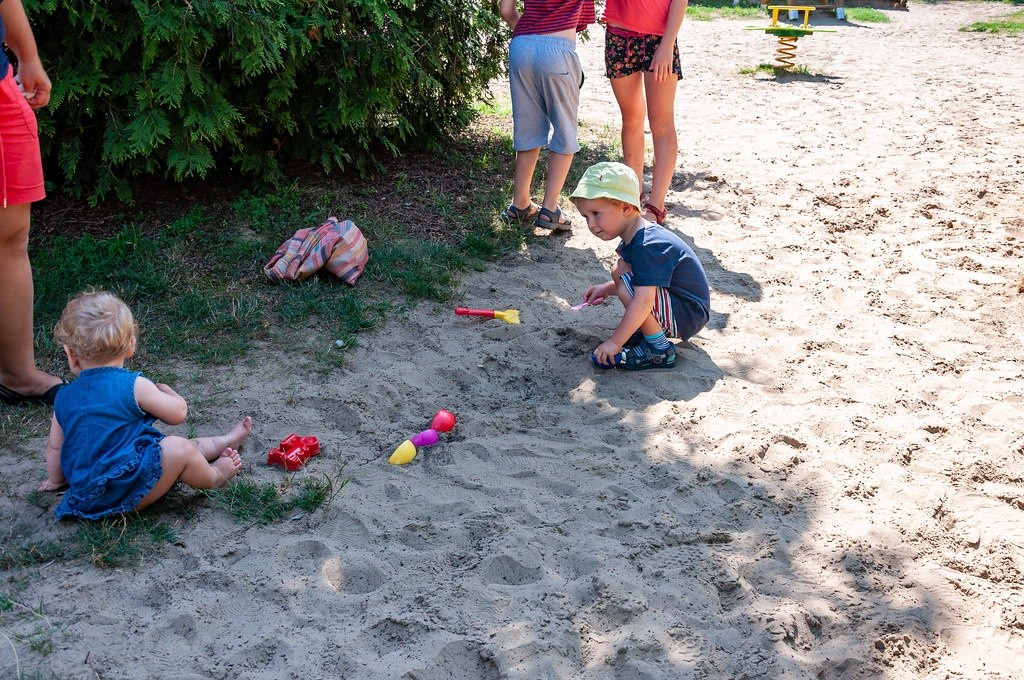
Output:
[0,376,69,404]
[640,191,668,227]
[534,206,573,231]
[502,200,540,224]
[613,331,676,370]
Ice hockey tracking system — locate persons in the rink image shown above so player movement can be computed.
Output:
[499,0,597,232]
[599,0,691,229]
[0,0,73,402]
[35,290,252,521]
[569,162,712,371]
